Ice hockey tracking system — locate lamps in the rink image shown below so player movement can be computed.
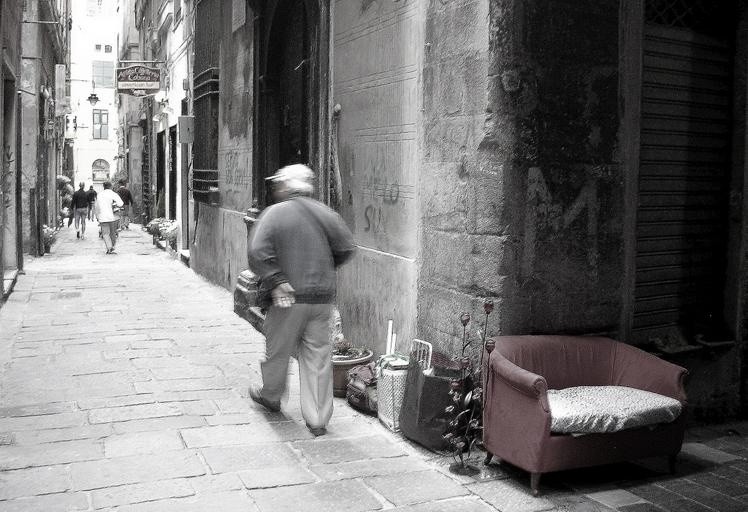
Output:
[67,78,100,107]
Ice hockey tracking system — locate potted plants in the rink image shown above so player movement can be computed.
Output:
[332,340,373,397]
[44,225,57,253]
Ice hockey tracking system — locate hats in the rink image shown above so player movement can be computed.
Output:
[265,164,314,186]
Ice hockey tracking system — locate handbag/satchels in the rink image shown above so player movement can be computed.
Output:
[375,351,411,434]
[345,364,378,415]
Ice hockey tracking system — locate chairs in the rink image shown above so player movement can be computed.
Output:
[483,336,690,497]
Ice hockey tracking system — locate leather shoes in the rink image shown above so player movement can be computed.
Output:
[305,422,327,437]
[248,384,281,413]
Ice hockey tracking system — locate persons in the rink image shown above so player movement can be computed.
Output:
[247,164,357,438]
[70,179,134,254]
